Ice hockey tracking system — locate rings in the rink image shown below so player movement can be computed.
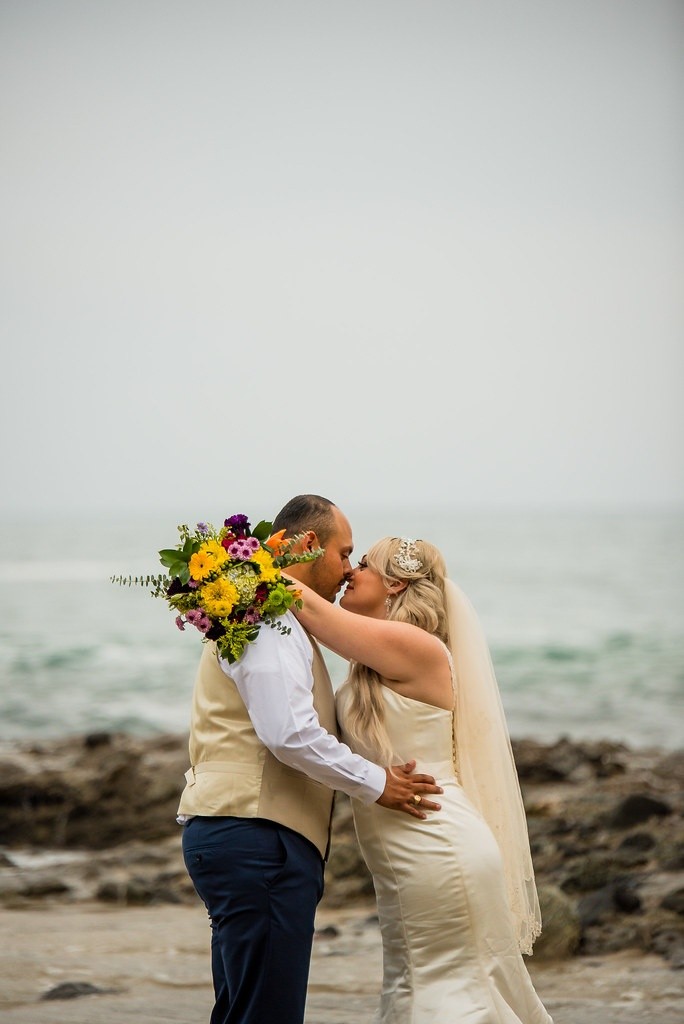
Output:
[414,795,421,805]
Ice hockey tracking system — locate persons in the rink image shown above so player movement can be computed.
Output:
[177,494,553,1024]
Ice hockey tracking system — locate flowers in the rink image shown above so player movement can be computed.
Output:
[108,512,325,662]
